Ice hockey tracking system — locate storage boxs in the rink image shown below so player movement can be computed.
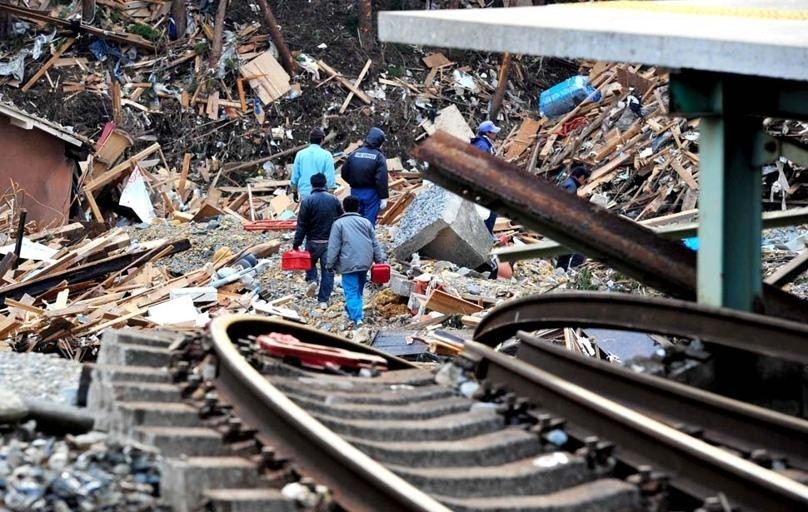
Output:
[280,248,311,271]
[370,263,391,284]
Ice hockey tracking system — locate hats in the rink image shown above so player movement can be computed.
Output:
[478,120,501,134]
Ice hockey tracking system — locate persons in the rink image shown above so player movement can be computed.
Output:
[556,166,591,271]
[468,121,502,236]
[293,173,345,309]
[326,195,381,330]
[290,127,338,205]
[341,126,389,230]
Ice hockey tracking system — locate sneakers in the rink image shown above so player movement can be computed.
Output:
[305,281,362,326]
